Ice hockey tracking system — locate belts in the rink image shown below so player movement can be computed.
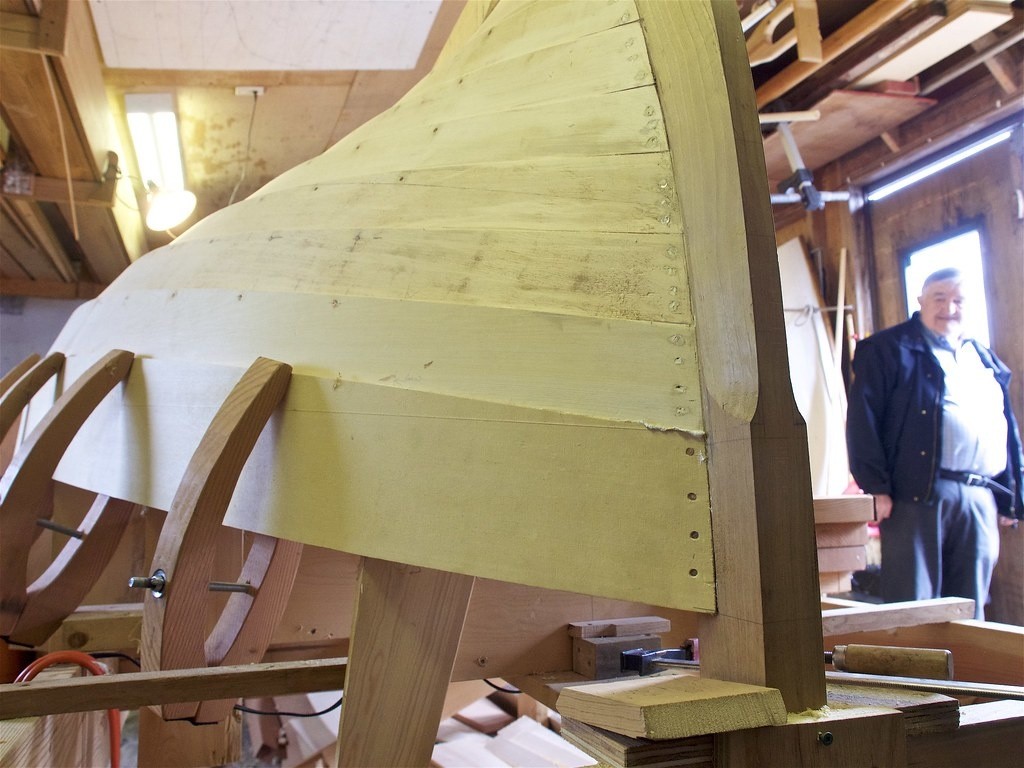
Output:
[939,469,1016,497]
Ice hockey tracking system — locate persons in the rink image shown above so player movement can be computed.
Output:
[846,268,1024,624]
